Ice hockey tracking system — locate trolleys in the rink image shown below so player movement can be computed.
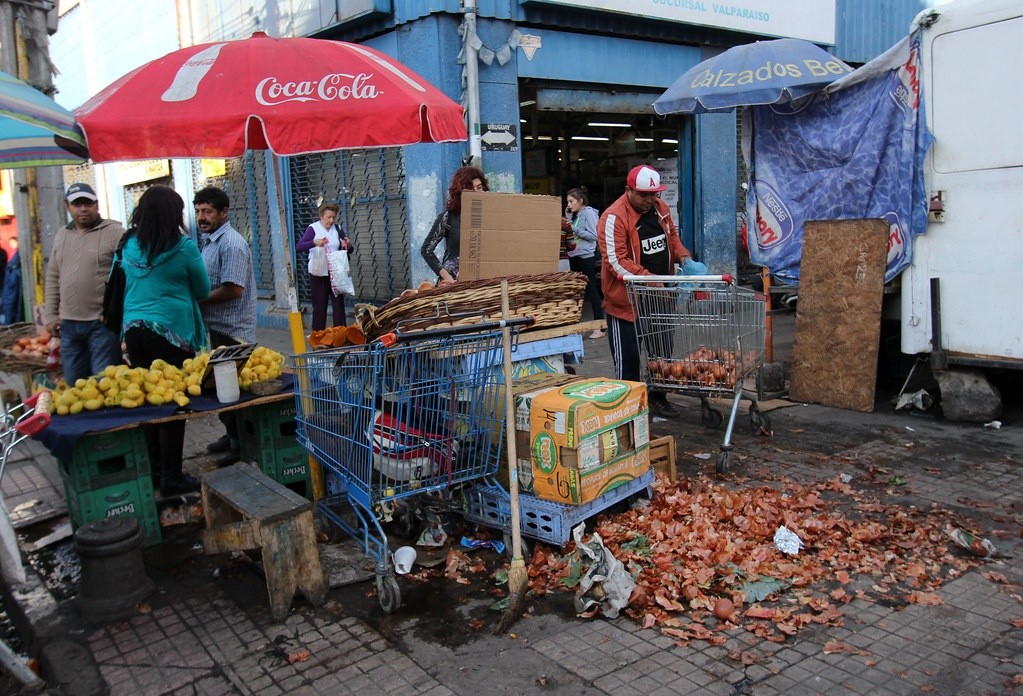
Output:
[289,301,536,616]
[614,273,770,475]
[0,392,61,696]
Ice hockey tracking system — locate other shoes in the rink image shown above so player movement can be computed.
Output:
[214,452,240,468]
[153,472,202,497]
[589,329,606,339]
[206,434,236,455]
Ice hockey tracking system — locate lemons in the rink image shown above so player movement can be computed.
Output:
[384,487,394,496]
[48,344,284,414]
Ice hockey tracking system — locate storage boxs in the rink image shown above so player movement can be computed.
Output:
[436,334,655,549]
[236,396,325,499]
[58,427,162,547]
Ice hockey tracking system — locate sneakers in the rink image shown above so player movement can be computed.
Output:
[648,395,681,417]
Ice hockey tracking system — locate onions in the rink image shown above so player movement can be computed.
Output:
[648,346,759,387]
[13,334,60,356]
[714,598,740,624]
[399,281,455,299]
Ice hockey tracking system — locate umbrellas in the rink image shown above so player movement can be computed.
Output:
[651,38,857,361]
[0,69,91,170]
[54,31,468,507]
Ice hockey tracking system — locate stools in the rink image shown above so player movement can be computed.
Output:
[201,462,327,623]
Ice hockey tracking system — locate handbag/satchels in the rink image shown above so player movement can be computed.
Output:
[324,239,356,298]
[103,228,138,335]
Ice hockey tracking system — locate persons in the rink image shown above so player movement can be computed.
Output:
[595,165,691,416]
[559,218,576,260]
[193,187,258,468]
[1,249,24,326]
[420,166,490,287]
[44,183,126,388]
[108,186,212,498]
[296,203,355,338]
[565,189,606,338]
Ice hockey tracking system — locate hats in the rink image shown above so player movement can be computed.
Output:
[627,164,667,192]
[64,183,98,202]
